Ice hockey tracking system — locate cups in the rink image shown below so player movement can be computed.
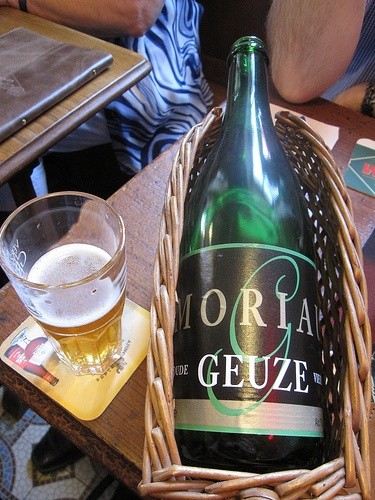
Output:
[0,191,125,377]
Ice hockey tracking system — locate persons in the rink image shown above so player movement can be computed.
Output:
[0,0,213,473]
[267,0,374,114]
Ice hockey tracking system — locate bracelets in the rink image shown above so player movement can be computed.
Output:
[360,79,375,119]
[19,0,27,12]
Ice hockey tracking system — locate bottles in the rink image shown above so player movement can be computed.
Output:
[174,36,324,472]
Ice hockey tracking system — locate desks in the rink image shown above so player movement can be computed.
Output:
[0,5,154,209]
[0,77,375,489]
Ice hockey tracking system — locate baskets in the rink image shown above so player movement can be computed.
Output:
[138,106,371,500]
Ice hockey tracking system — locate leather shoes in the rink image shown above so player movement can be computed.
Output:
[32,426,86,474]
